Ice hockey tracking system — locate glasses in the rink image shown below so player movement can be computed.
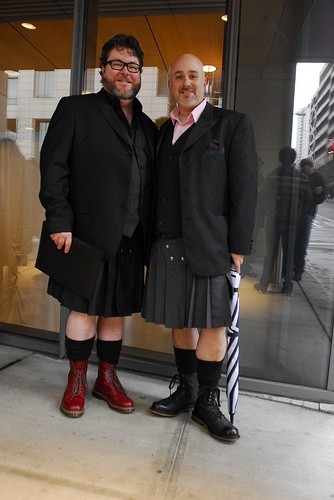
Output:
[103,59,141,73]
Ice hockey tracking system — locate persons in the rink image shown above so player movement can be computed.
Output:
[142,54,258,442]
[241,148,328,296]
[36,34,157,418]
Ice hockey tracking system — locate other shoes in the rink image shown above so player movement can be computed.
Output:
[254,279,268,293]
[282,280,293,295]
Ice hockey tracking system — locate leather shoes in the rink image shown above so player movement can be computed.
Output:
[60,361,88,417]
[149,373,199,416]
[191,392,241,441]
[92,362,134,413]
